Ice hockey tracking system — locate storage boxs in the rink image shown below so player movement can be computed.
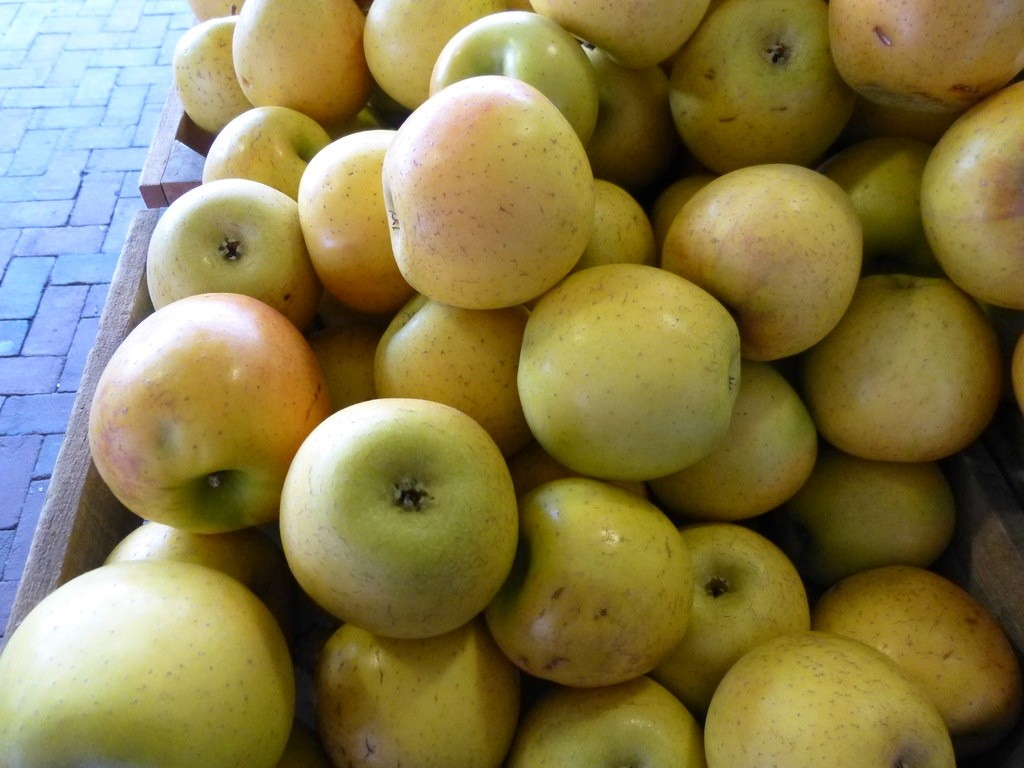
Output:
[4,1,1024,767]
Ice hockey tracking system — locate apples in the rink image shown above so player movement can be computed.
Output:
[0,0,1024,768]
